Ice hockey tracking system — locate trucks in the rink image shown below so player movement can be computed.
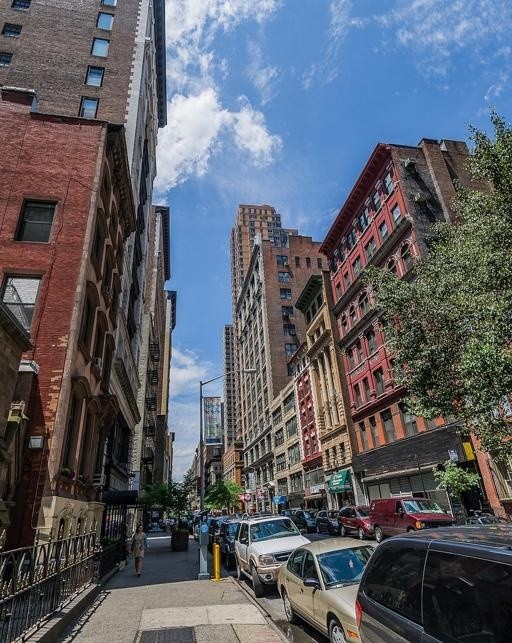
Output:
[369,496,453,543]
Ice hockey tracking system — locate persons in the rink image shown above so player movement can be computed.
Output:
[129,522,151,578]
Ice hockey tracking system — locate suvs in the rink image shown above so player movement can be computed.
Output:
[233,511,312,598]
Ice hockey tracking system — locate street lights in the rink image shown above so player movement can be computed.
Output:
[199,368,259,545]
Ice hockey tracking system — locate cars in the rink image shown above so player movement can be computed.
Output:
[354,524,512,642]
[277,538,380,643]
[208,515,239,569]
[281,507,372,540]
